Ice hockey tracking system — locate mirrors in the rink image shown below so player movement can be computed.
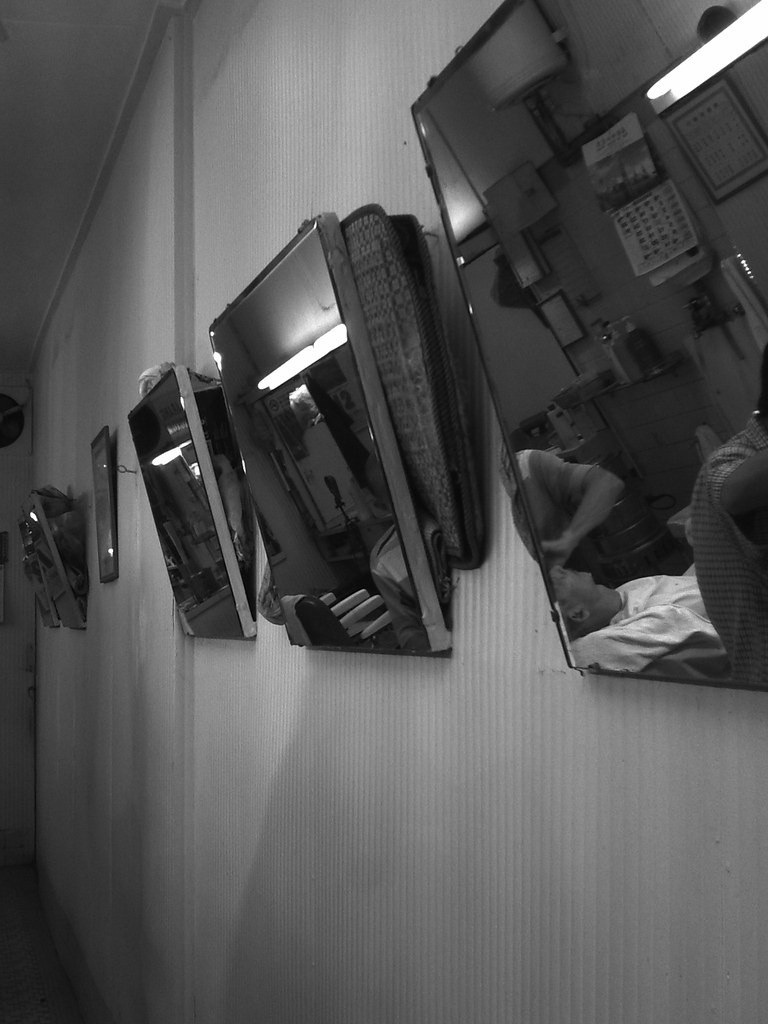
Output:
[16,515,61,630]
[410,0,767,697]
[20,491,88,631]
[203,207,455,665]
[124,360,259,644]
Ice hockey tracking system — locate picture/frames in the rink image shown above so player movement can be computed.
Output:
[89,425,121,585]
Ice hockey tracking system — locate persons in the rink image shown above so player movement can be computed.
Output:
[513,445,626,573]
[687,341,768,692]
[547,563,734,683]
[28,560,50,612]
